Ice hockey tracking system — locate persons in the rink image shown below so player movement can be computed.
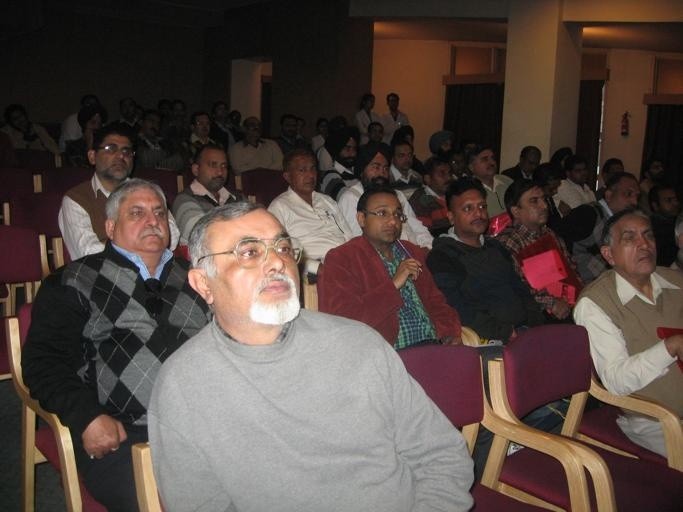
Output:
[574,205,682,457]
[145,202,477,510]
[21,178,213,511]
[0,82,680,435]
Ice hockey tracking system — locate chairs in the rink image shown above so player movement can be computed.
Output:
[480,324,683,511]
[397,344,589,512]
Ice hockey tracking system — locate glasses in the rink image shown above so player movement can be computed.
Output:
[97,144,135,157]
[197,237,303,268]
[361,208,406,223]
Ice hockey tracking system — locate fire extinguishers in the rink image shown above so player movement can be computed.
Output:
[621,112,631,136]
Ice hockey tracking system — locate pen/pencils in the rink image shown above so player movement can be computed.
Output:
[397,238,423,273]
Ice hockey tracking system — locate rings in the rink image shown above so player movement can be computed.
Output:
[109,446,122,452]
